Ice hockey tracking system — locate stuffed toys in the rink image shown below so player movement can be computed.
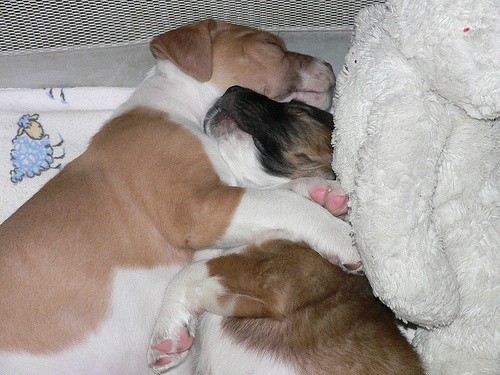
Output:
[331,0,500,375]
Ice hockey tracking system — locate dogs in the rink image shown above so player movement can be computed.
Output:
[146,85,428,375]
[1,19,336,375]
